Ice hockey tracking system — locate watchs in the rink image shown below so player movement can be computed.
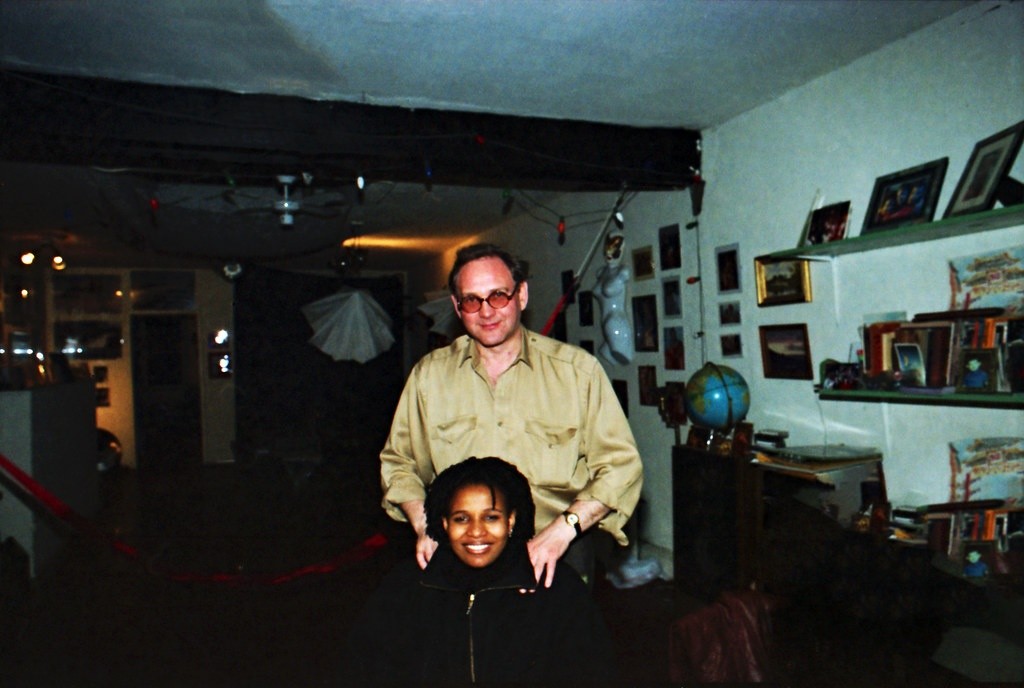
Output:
[561,510,582,535]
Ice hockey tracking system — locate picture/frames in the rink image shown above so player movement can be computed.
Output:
[209,331,234,379]
[558,223,690,424]
[714,199,851,381]
[0,267,124,408]
[861,121,1024,236]
[864,308,1024,395]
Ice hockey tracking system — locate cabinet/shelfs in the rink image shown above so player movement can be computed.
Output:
[670,445,1024,688]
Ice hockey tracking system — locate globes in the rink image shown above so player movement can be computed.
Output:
[684,361,751,443]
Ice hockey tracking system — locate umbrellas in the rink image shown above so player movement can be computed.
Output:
[416,289,462,338]
[301,286,395,364]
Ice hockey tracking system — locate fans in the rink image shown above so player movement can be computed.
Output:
[215,175,339,227]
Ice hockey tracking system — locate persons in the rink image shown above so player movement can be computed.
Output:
[380,456,615,688]
[965,551,989,575]
[669,328,683,368]
[49,352,74,381]
[721,306,739,323]
[894,187,920,209]
[724,336,740,353]
[720,259,732,290]
[903,356,911,368]
[591,232,631,366]
[637,302,654,346]
[336,244,644,688]
[965,358,988,388]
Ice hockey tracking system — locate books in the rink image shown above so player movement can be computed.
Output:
[847,481,1007,557]
[900,321,955,386]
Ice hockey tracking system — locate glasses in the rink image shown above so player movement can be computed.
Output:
[457,284,520,313]
